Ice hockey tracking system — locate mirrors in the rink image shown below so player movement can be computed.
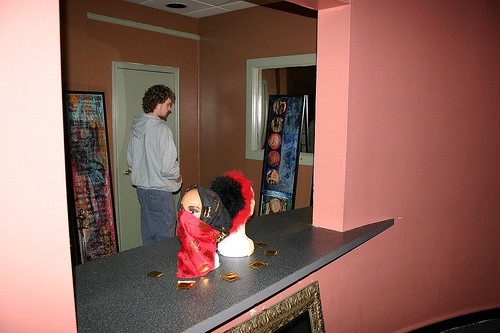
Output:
[261,65,316,153]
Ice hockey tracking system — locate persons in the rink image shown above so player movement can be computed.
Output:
[126,84,182,246]
[209,170,255,257]
[176,184,233,277]
